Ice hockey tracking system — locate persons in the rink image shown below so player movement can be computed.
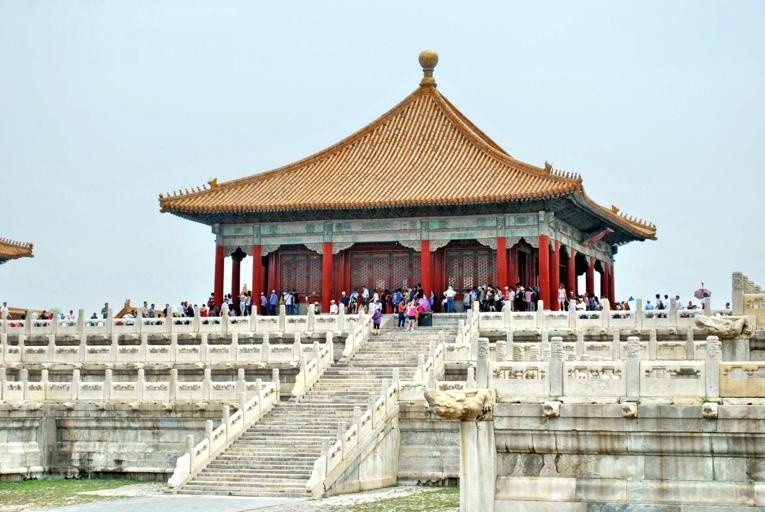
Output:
[260,289,299,315]
[67,303,108,326]
[314,302,320,314]
[557,284,670,319]
[675,293,710,317]
[463,283,539,311]
[240,291,251,316]
[2,302,12,319]
[20,310,53,326]
[176,293,234,325]
[370,283,436,334]
[441,286,457,313]
[123,301,169,325]
[330,286,369,315]
[725,302,730,309]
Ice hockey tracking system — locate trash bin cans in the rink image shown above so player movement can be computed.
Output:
[418,312,432,326]
